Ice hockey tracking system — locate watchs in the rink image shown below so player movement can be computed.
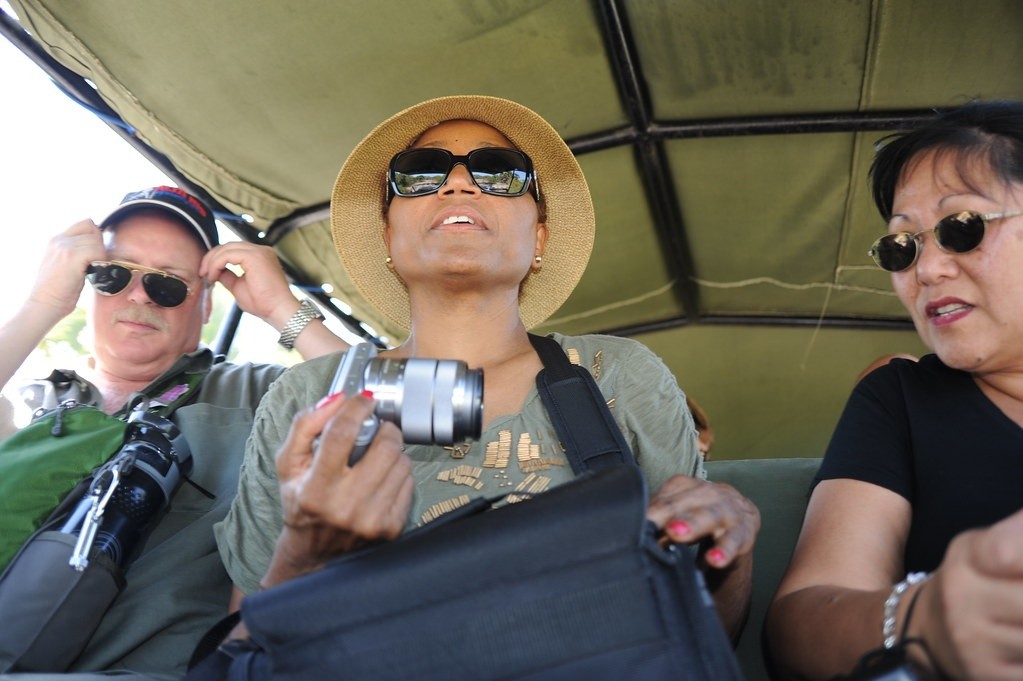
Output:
[278,299,326,351]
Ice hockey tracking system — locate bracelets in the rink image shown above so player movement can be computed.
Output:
[882,572,926,648]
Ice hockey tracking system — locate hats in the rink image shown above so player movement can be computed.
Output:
[329,96,596,331]
[97,186,221,250]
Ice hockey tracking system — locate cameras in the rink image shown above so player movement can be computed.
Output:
[311,342,484,469]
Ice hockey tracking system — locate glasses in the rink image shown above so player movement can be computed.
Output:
[85,260,205,309]
[868,210,1023,272]
[385,148,541,206]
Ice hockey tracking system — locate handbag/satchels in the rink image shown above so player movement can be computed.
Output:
[237,461,743,681]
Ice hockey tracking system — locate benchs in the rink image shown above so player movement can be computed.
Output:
[703,456,822,681]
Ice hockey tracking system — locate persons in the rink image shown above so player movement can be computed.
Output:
[767,100,1023,681]
[0,186,351,681]
[215,96,759,680]
